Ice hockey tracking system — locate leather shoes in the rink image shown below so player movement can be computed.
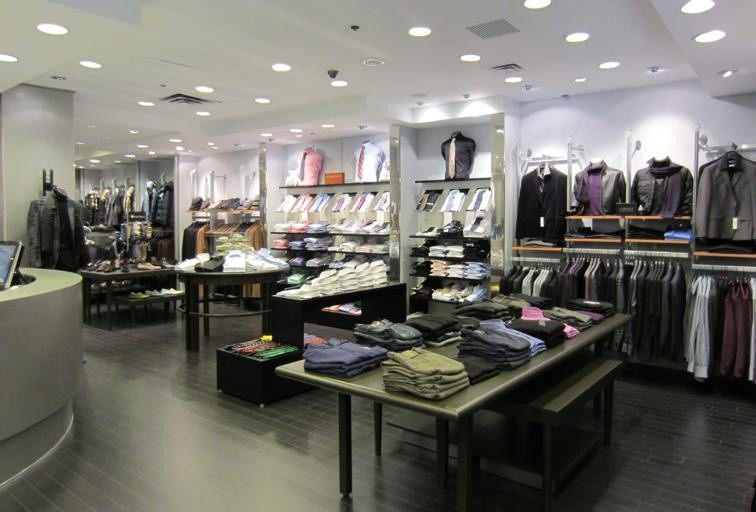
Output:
[90,279,132,291]
[127,288,183,299]
[82,255,176,273]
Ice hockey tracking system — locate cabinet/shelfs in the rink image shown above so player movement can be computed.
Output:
[503,125,756,386]
[182,195,261,259]
[269,141,389,331]
[27,169,175,270]
[408,133,492,319]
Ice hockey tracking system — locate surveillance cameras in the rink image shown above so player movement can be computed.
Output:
[328,70,338,78]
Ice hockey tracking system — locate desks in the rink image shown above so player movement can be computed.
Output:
[217,275,406,406]
[78,259,290,352]
[274,291,634,510]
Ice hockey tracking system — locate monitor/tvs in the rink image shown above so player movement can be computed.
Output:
[0,240,28,290]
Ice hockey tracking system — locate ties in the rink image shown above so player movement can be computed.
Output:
[313,195,323,211]
[334,198,344,211]
[445,192,455,211]
[358,144,365,179]
[448,138,456,179]
[355,192,368,212]
[471,217,483,230]
[473,190,485,210]
[299,151,307,181]
[419,192,430,210]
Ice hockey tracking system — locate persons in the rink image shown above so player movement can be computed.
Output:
[630,151,691,216]
[571,157,627,215]
[441,132,476,180]
[352,139,387,183]
[26,188,92,274]
[296,146,322,186]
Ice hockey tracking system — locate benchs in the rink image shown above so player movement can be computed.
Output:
[436,353,627,508]
